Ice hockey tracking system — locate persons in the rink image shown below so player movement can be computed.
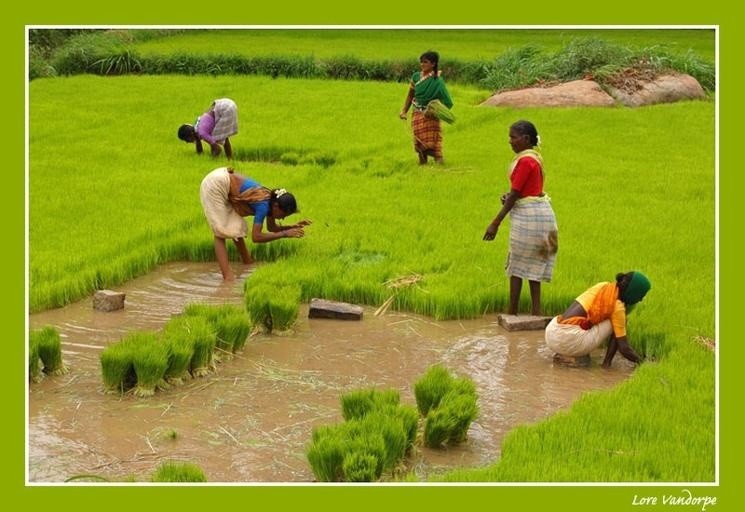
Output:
[541,268,652,372]
[177,97,240,160]
[197,164,311,284]
[398,48,455,165]
[482,120,559,318]
